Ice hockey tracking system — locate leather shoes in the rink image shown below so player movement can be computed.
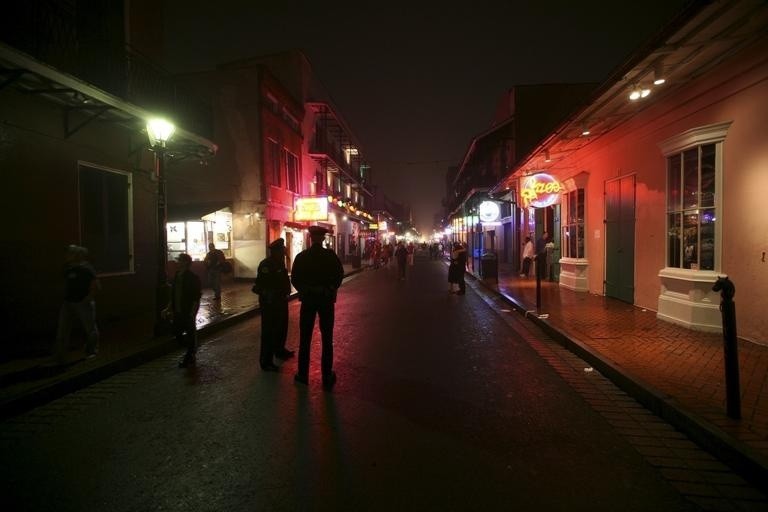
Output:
[263,363,278,371]
[276,347,295,358]
[323,371,335,389]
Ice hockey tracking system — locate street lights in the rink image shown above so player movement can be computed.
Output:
[142,115,181,333]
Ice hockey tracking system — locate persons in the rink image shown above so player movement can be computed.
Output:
[252,237,295,371]
[163,253,203,368]
[324,238,468,296]
[519,236,534,278]
[291,226,344,386]
[40,244,100,368]
[544,236,555,281]
[534,231,550,280]
[203,243,227,300]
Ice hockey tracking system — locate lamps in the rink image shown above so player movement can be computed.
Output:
[653,63,675,85]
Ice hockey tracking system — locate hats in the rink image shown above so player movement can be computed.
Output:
[308,226,328,239]
[268,238,285,249]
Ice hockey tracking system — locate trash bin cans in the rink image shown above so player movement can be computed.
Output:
[480,253,499,279]
